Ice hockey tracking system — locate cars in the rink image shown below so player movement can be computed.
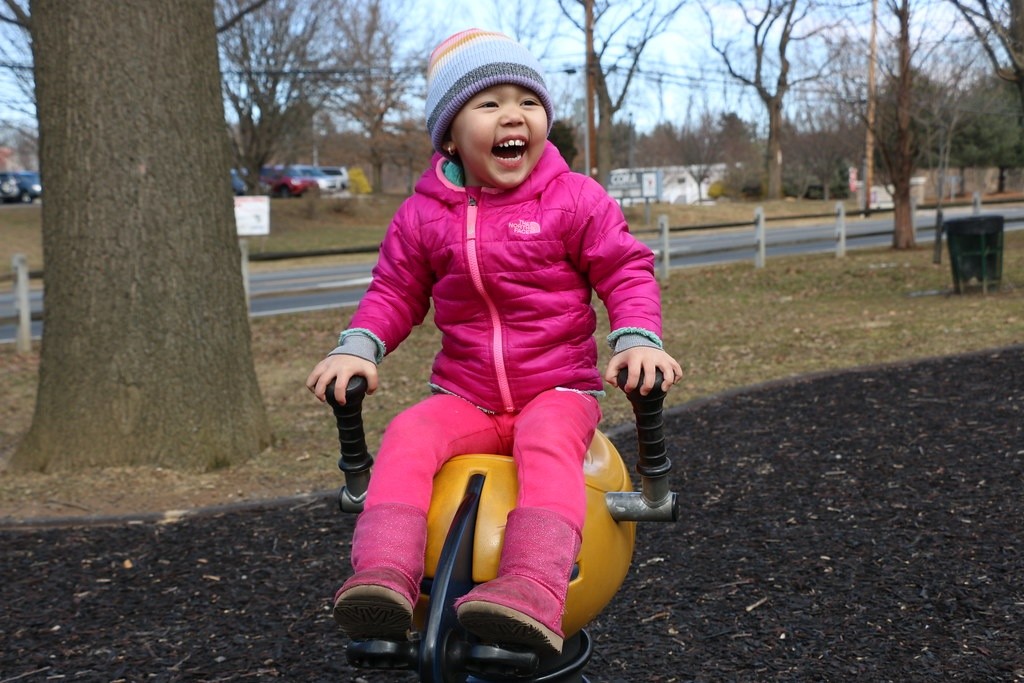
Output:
[0,170,41,203]
[231,164,350,197]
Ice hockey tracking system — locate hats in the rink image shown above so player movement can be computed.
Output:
[425,28,554,167]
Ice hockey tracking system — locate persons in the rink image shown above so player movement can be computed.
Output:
[301,24,684,656]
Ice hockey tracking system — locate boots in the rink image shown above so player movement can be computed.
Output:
[332,502,429,643]
[453,507,583,657]
